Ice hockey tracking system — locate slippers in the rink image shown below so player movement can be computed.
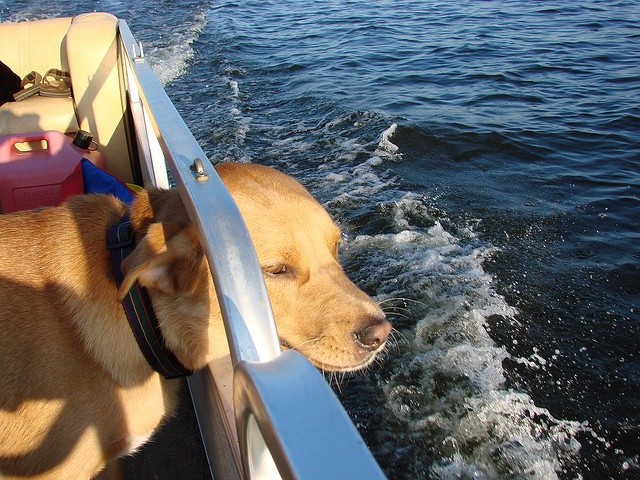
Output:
[13,71,42,101]
[40,68,72,98]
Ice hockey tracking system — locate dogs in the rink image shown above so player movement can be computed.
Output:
[1,163,427,479]
